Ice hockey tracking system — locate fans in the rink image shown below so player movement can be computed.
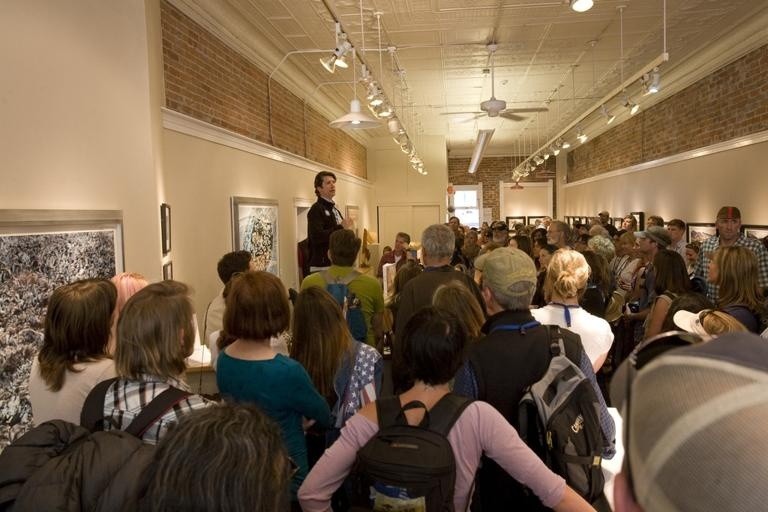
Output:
[439,41,549,128]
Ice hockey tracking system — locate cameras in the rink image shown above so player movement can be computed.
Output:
[627,301,640,315]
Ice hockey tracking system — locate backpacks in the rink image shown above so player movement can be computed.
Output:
[317,269,368,346]
[516,322,616,503]
[340,390,477,512]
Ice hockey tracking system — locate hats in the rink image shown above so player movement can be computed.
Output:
[473,246,538,297]
[610,331,767,510]
[598,210,610,216]
[717,206,740,220]
[633,226,672,248]
[672,308,732,344]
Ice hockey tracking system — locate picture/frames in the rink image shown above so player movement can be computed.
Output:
[230,195,279,284]
[163,260,173,281]
[344,205,360,229]
[506,212,768,245]
[0,208,125,455]
[161,203,171,253]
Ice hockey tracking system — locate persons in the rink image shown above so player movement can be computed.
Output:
[30,208,767,512]
[305,172,354,274]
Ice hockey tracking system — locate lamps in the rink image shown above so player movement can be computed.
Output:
[511,0,668,183]
[467,130,495,175]
[319,0,428,175]
